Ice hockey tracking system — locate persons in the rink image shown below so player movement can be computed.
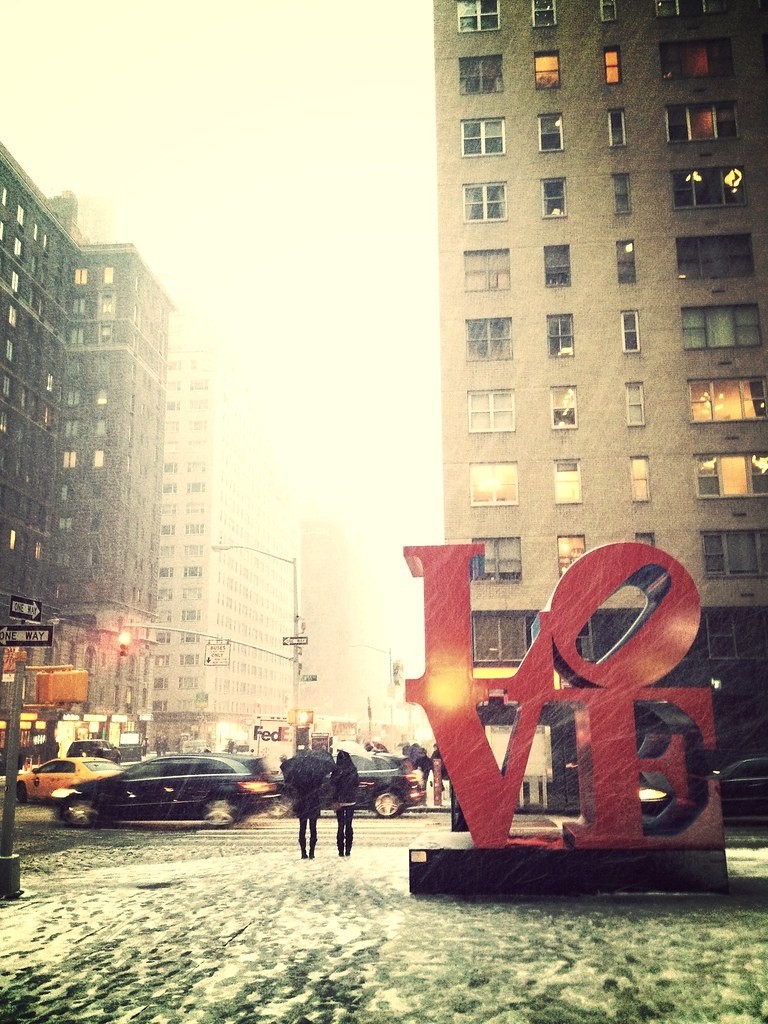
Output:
[141,735,183,757]
[292,749,327,859]
[330,751,360,857]
[364,741,450,806]
[228,738,235,753]
[66,746,123,765]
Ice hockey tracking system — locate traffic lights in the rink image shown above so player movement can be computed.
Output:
[118,631,132,657]
[287,708,314,727]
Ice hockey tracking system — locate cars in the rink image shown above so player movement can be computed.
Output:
[50,753,285,830]
[268,752,428,819]
[14,757,128,805]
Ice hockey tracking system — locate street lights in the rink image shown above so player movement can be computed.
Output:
[350,643,394,754]
[209,543,302,756]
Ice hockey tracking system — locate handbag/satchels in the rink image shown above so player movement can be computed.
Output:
[331,802,341,813]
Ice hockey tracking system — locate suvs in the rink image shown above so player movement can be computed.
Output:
[65,739,123,766]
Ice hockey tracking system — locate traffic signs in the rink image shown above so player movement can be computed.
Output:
[0,624,54,647]
[205,643,232,667]
[282,636,309,646]
[301,674,317,682]
[9,594,44,625]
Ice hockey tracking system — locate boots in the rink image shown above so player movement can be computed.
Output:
[309,838,317,859]
[298,838,308,858]
